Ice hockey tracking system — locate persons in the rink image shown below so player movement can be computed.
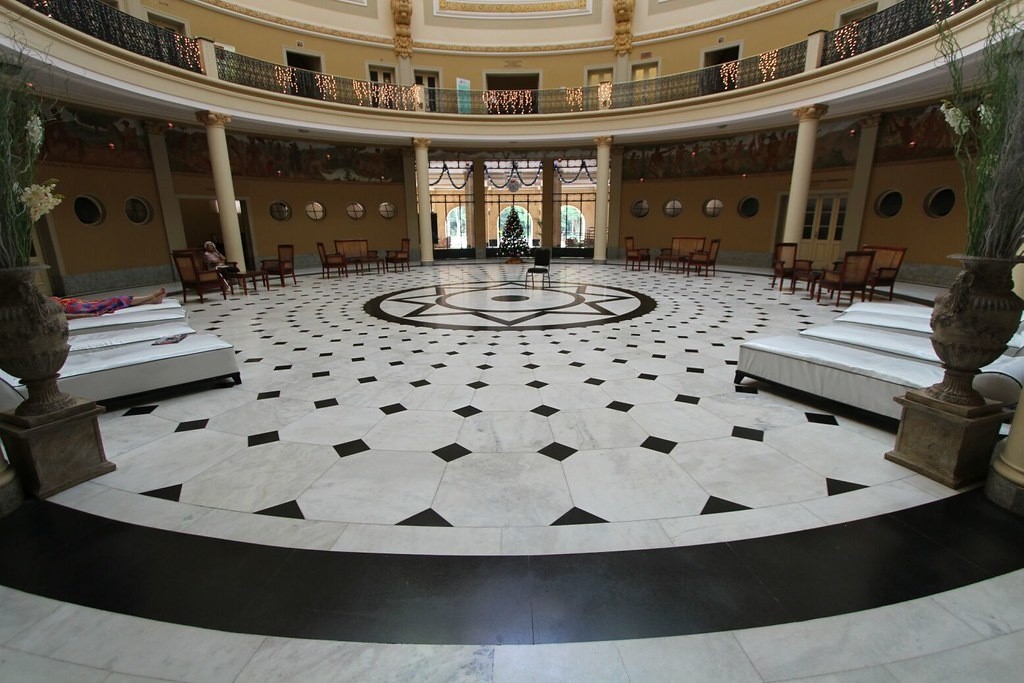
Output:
[48,287,166,320]
[203,241,248,293]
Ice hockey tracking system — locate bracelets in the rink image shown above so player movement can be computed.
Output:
[218,252,220,255]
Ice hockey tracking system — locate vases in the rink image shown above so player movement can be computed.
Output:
[0,261,79,418]
[925,253,1024,406]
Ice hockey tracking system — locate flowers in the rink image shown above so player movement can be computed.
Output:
[925,0,1024,258]
[0,6,69,269]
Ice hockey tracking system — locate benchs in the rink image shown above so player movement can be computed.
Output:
[172,249,240,294]
[659,235,708,272]
[826,243,908,302]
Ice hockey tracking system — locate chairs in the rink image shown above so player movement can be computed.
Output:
[624,236,651,272]
[261,243,297,287]
[687,239,723,277]
[735,302,1024,425]
[771,242,814,292]
[0,297,247,416]
[317,243,350,279]
[386,238,412,273]
[524,247,552,290]
[816,248,878,306]
[172,254,229,303]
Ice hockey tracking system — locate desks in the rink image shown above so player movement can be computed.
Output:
[354,257,386,275]
[334,239,379,271]
[654,253,686,274]
[792,269,833,301]
[230,269,270,296]
